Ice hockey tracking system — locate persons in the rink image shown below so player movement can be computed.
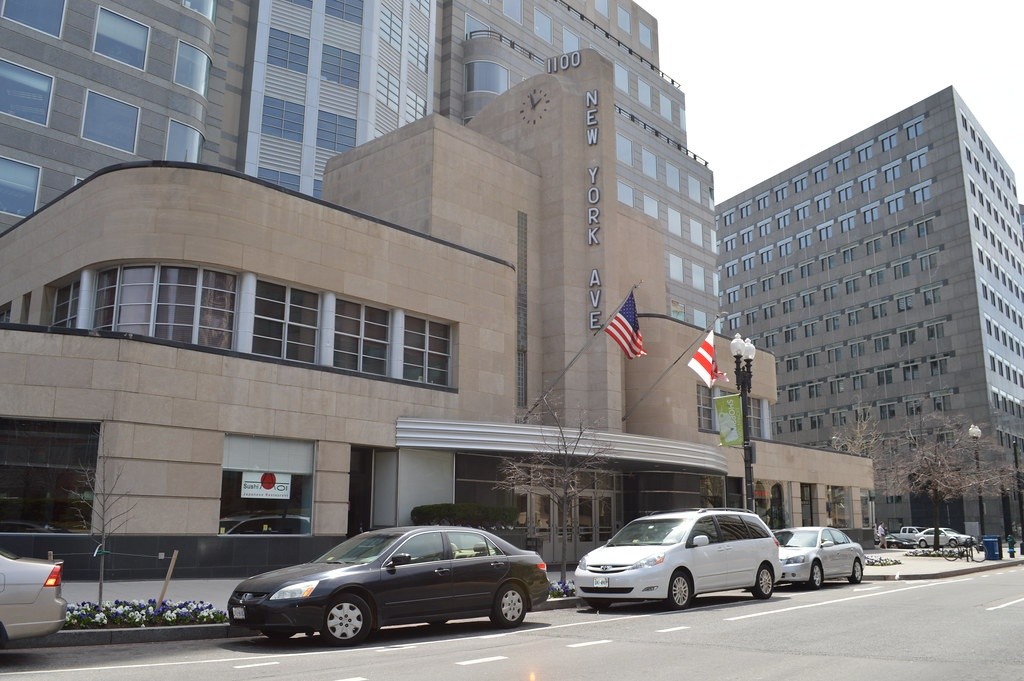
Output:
[878,521,887,549]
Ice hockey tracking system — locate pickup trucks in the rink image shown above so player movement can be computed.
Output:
[884,526,927,549]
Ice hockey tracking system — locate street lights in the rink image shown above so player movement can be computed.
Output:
[730,333,757,513]
[968,424,986,535]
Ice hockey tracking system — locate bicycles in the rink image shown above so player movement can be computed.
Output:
[941,534,988,563]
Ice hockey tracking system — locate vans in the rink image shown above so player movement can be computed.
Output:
[573,506,784,610]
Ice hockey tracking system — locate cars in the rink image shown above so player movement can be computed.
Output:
[772,526,866,589]
[225,524,552,647]
[0,547,67,650]
[914,527,979,549]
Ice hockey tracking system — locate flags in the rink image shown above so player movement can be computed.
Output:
[687,330,729,389]
[603,290,647,360]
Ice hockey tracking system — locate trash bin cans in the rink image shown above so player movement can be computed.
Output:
[982,538,999,560]
[526,538,544,560]
